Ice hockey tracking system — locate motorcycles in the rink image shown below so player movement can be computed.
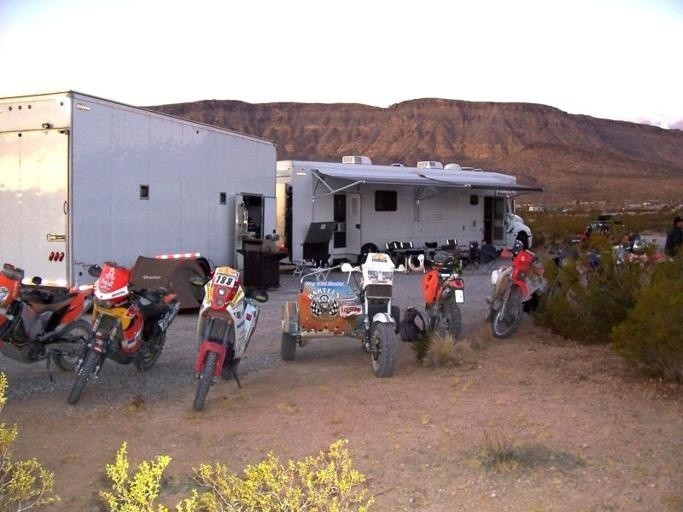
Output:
[406,247,469,348]
[1,263,93,386]
[64,260,177,408]
[481,217,674,339]
[277,253,402,379]
[182,256,270,415]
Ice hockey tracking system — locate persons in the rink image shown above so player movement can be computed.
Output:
[665,217,683,255]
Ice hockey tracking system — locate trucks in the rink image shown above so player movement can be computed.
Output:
[276,153,540,269]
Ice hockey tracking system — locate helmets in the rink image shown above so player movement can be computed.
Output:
[433,250,453,273]
